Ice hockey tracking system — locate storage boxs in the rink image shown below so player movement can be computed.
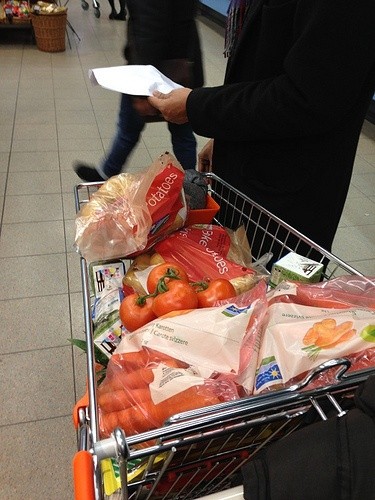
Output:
[28,10,67,53]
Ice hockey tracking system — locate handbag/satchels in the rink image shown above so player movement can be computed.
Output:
[122,222,271,293]
[133,58,201,123]
[252,273,375,398]
[98,280,273,500]
[70,149,188,267]
[182,192,221,227]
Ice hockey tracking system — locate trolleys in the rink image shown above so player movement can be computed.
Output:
[71,166,373,499]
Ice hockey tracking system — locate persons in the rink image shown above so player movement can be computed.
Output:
[107,0,127,21]
[71,0,205,184]
[147,1,374,283]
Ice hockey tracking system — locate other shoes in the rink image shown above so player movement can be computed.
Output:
[109,10,118,19]
[116,10,127,20]
[74,163,105,183]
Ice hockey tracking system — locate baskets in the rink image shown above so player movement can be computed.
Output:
[13,16,30,25]
[30,7,67,53]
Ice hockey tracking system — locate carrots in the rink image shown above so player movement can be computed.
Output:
[299,318,356,361]
[96,349,222,439]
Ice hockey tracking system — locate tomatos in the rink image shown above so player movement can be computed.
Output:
[361,325,375,343]
[118,263,238,334]
